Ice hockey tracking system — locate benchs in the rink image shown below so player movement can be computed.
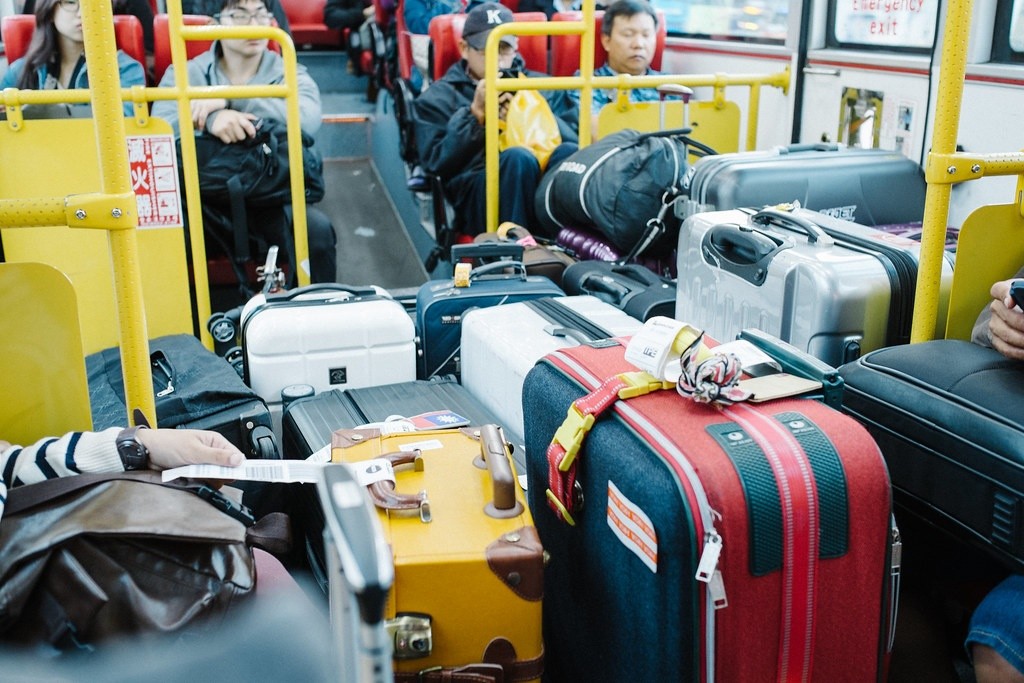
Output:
[277,0,351,51]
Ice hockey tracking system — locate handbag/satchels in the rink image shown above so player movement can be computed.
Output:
[495,74,562,169]
[834,334,1022,574]
[0,466,290,664]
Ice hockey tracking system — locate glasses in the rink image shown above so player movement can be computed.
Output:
[213,11,273,25]
[56,0,79,11]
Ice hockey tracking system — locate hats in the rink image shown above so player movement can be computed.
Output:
[463,2,519,50]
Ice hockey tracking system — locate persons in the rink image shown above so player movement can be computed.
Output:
[323,0,376,29]
[0,426,330,644]
[151,0,338,290]
[404,0,499,93]
[410,3,580,237]
[567,0,682,142]
[964,266,1024,683]
[0,0,146,119]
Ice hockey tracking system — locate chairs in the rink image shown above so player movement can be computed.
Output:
[551,8,667,78]
[153,13,337,288]
[1,14,151,114]
[360,0,548,264]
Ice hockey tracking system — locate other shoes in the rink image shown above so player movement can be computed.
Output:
[406,164,431,192]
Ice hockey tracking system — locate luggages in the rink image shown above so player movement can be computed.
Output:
[85,143,1023,683]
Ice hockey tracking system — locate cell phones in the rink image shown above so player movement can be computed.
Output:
[498,69,518,104]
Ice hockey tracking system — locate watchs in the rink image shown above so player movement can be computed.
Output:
[116,425,150,470]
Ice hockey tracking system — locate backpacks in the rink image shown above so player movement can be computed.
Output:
[177,118,325,263]
[535,129,717,264]
